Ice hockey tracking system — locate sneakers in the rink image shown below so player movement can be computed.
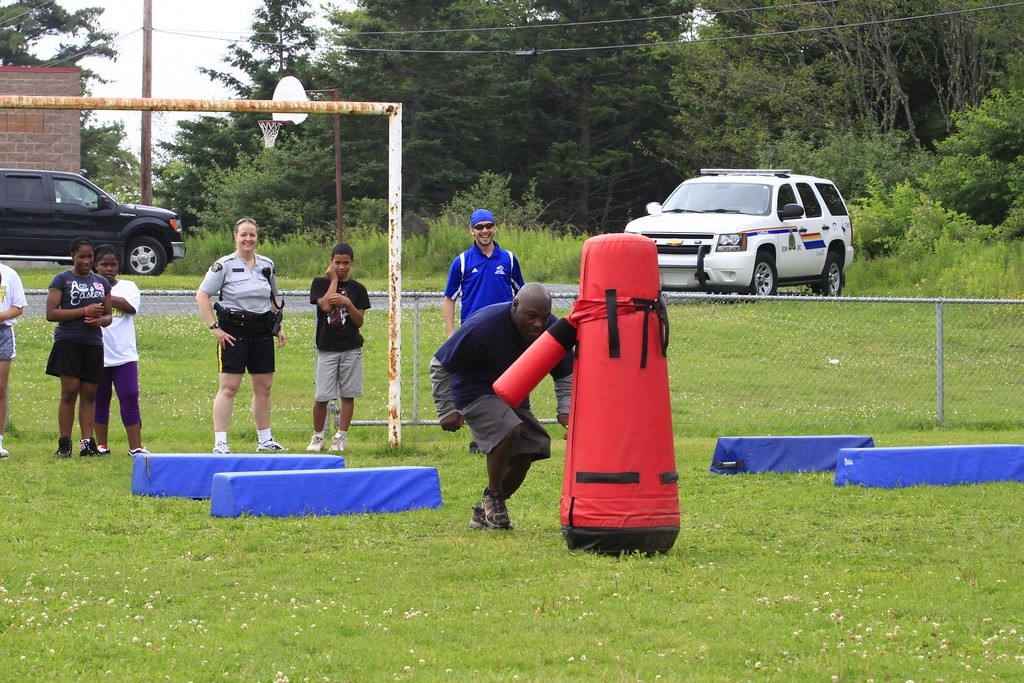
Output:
[54,437,73,460]
[256,439,289,452]
[469,506,512,533]
[328,432,348,452]
[213,441,233,455]
[128,444,150,456]
[306,432,325,453]
[482,488,509,529]
[97,444,111,454]
[79,437,109,458]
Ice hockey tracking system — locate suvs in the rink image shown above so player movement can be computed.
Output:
[623,168,856,303]
[0,168,188,278]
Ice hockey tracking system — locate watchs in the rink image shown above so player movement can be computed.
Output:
[210,322,220,329]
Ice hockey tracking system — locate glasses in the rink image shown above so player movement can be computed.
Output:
[235,218,256,226]
[473,223,494,230]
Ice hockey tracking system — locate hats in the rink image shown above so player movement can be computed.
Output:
[470,209,495,227]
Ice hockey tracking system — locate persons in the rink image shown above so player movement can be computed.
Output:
[44,237,113,459]
[306,244,372,452]
[443,209,525,454]
[0,261,28,457]
[195,216,287,455]
[91,244,152,457]
[428,282,575,530]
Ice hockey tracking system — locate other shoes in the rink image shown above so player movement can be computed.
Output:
[469,440,480,454]
[0,448,10,460]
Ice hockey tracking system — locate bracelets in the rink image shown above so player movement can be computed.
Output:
[84,307,88,316]
